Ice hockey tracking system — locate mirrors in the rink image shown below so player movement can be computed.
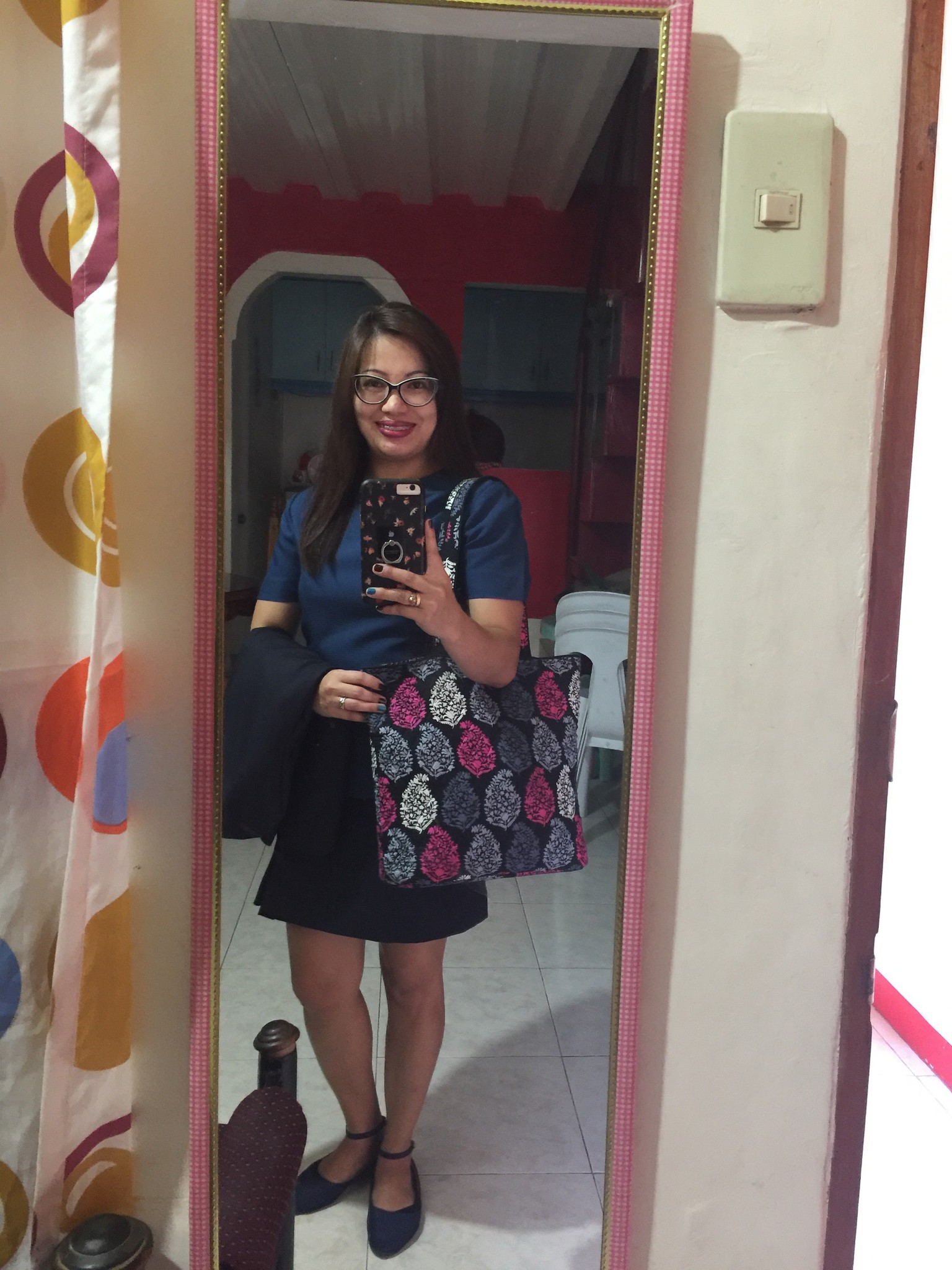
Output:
[186,0,694,1270]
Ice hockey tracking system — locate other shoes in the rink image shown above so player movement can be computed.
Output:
[293,1117,387,1217]
[366,1142,424,1259]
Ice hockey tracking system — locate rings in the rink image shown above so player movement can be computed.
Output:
[339,697,348,710]
[409,591,421,607]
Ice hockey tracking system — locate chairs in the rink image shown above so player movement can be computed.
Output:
[554,590,631,816]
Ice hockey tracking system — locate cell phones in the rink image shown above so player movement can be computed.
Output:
[360,479,425,604]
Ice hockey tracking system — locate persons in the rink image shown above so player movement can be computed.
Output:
[247,301,530,1256]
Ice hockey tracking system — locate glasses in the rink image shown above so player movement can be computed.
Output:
[352,373,441,406]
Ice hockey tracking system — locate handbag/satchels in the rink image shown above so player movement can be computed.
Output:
[360,476,589,885]
